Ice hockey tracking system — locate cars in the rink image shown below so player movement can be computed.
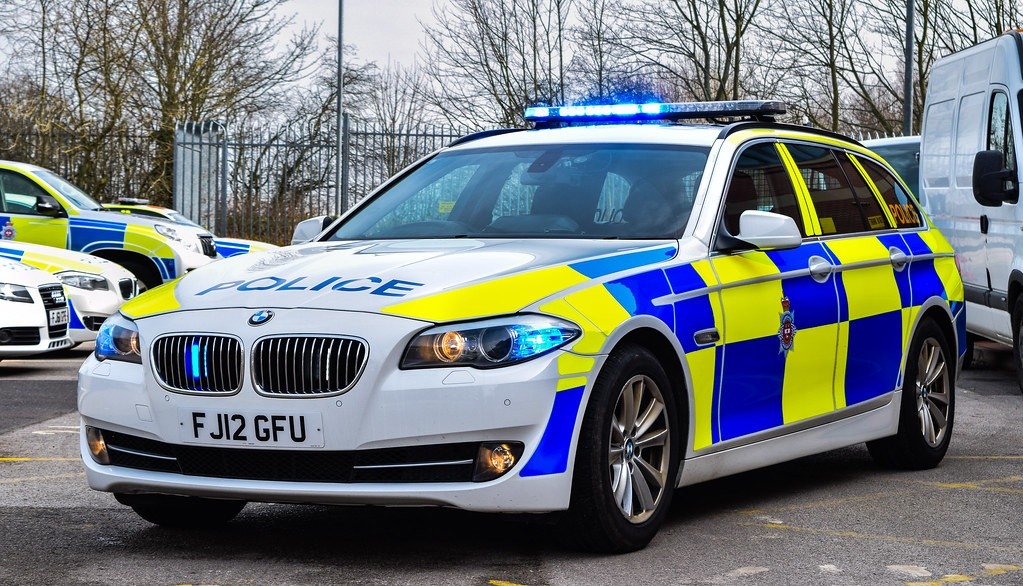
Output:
[0,256,76,364]
[0,238,148,352]
[77,99,968,559]
[0,160,281,291]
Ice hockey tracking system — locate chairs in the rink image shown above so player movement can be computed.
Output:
[692,169,758,235]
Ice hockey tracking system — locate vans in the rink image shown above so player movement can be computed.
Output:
[916,28,1023,399]
[842,134,922,204]
